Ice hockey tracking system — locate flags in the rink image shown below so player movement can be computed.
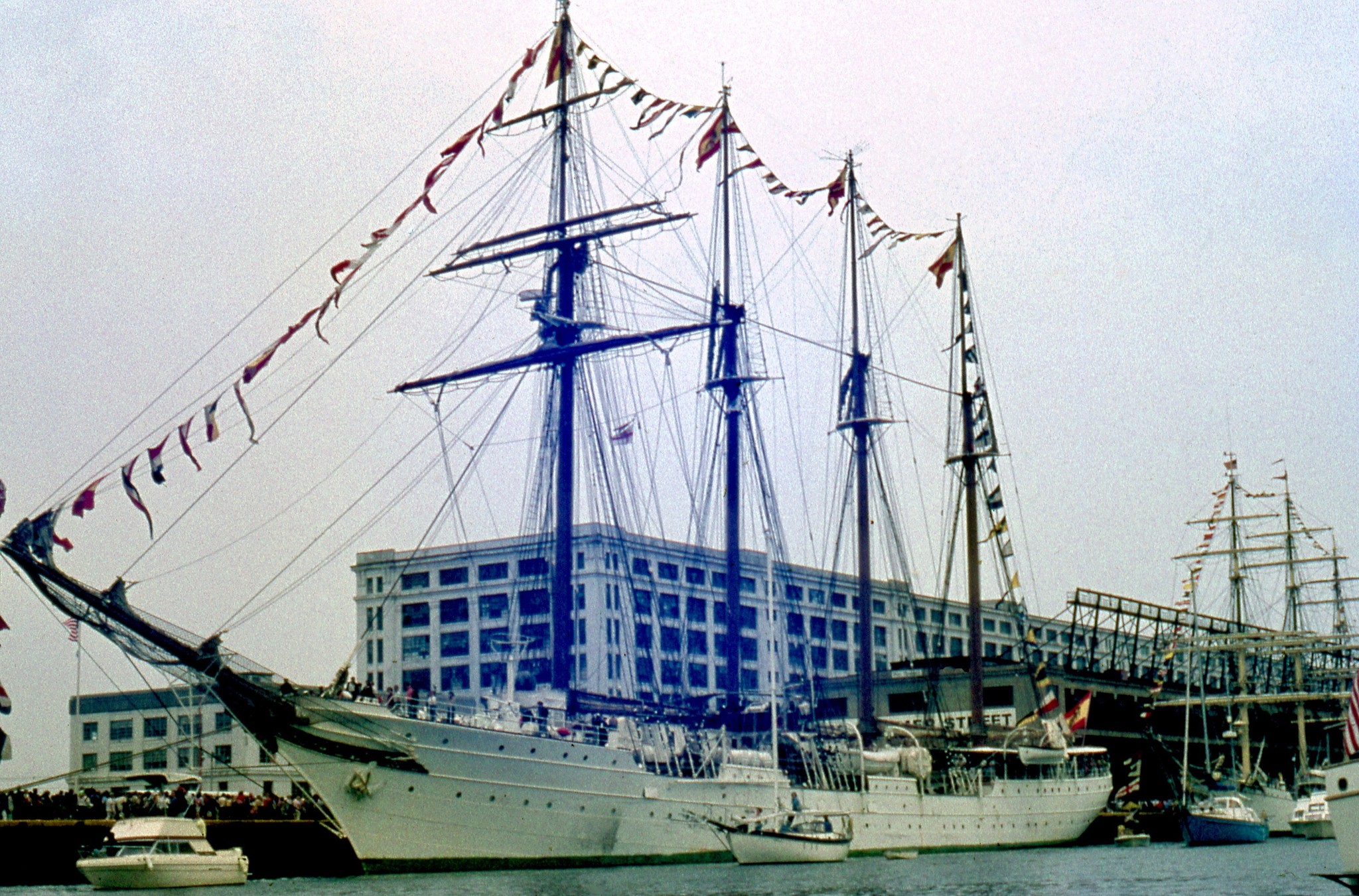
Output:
[1059,692,1090,732]
[1342,671,1359,754]
[0,615,13,760]
[423,38,549,213]
[1114,754,1174,810]
[961,321,998,474]
[612,422,634,445]
[65,618,79,642]
[545,18,703,138]
[1290,503,1322,550]
[696,112,797,197]
[240,200,420,382]
[826,165,944,258]
[1187,485,1229,573]
[1142,574,1199,717]
[986,486,1059,718]
[927,240,970,316]
[46,383,258,549]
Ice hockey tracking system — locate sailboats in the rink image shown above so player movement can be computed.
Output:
[679,538,856,865]
[1140,450,1359,896]
[0,1,1115,874]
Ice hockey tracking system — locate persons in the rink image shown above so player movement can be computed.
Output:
[0,787,334,821]
[280,676,454,722]
[518,702,617,746]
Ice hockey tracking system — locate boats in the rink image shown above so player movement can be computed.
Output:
[1016,744,1067,767]
[812,738,902,777]
[76,771,252,889]
